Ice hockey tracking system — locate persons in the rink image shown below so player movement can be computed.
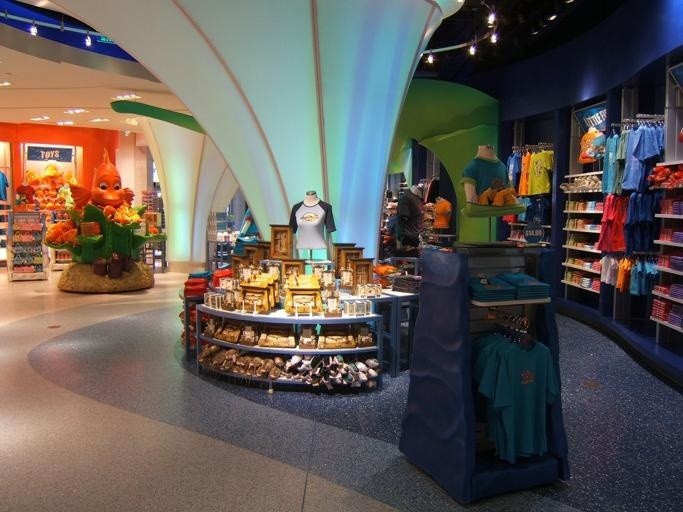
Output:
[240,208,258,236]
[459,145,510,205]
[16,181,35,203]
[432,197,452,230]
[287,191,337,251]
[397,184,425,247]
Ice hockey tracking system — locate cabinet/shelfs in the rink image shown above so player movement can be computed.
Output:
[6,209,72,282]
[559,157,683,343]
[177,288,420,393]
